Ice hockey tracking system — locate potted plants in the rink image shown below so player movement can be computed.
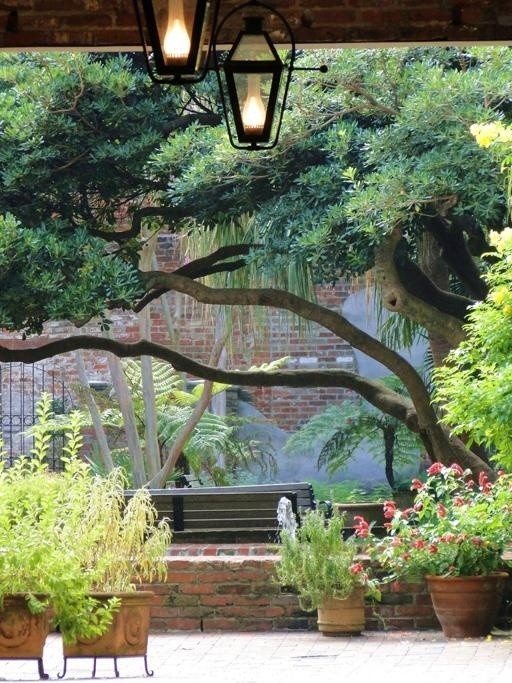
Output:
[61,467,172,677]
[4,390,84,679]
[267,491,386,637]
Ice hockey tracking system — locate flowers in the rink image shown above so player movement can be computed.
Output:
[345,458,512,604]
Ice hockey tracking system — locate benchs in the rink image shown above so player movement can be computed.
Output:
[119,479,346,545]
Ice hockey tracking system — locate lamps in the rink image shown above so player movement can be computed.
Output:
[131,0,328,153]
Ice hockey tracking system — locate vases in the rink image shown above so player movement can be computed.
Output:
[423,572,510,641]
